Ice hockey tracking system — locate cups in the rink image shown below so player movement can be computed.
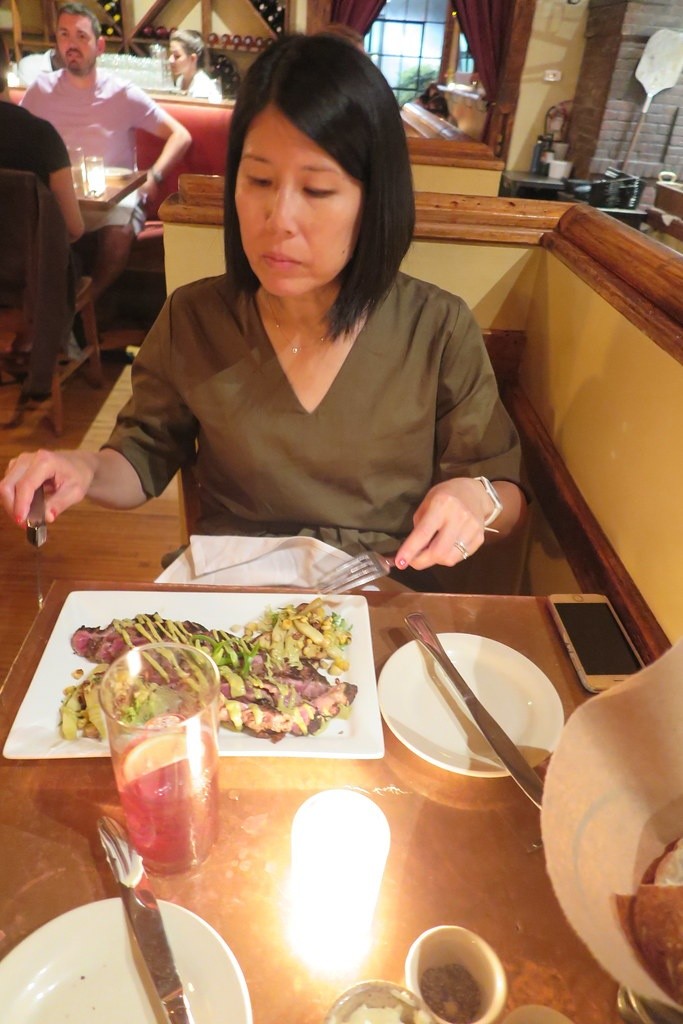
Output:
[66,143,85,199]
[402,924,509,1024]
[99,642,219,878]
[84,145,106,198]
[323,979,438,1024]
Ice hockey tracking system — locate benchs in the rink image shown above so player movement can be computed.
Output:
[9,85,239,272]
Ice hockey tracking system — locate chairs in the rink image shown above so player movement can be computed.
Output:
[0,169,103,437]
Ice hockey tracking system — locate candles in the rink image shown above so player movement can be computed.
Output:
[84,154,105,195]
[283,789,390,958]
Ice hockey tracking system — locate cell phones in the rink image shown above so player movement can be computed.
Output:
[547,594,646,693]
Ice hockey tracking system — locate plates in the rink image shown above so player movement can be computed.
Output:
[2,590,385,760]
[0,895,253,1024]
[378,632,564,779]
[503,1002,575,1024]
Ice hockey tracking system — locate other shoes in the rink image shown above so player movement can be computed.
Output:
[100,348,135,364]
[73,312,88,351]
[6,339,34,375]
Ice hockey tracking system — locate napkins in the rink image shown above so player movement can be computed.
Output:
[154,535,381,591]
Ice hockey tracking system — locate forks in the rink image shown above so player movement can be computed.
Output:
[317,551,396,595]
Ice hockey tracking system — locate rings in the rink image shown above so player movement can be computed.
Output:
[453,541,470,559]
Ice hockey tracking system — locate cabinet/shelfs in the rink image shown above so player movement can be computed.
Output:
[0,0,291,100]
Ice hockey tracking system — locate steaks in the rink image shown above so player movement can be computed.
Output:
[73,615,359,739]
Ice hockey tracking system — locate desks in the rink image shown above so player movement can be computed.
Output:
[497,170,566,199]
[70,164,147,213]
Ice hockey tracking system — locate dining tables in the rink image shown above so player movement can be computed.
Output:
[0,580,645,1024]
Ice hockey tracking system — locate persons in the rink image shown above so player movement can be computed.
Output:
[0,6,192,367]
[0,32,533,592]
[415,84,449,119]
[168,29,222,102]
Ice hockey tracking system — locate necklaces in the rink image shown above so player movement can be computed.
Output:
[267,299,328,354]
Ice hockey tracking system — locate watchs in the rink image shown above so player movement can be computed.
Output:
[470,475,504,534]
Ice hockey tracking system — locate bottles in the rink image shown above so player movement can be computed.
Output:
[97,0,286,98]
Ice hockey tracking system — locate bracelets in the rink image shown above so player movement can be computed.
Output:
[151,166,164,184]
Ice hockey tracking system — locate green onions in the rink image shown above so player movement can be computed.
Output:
[187,634,262,675]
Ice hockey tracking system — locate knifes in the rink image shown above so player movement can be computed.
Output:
[403,612,543,809]
[95,814,193,1024]
